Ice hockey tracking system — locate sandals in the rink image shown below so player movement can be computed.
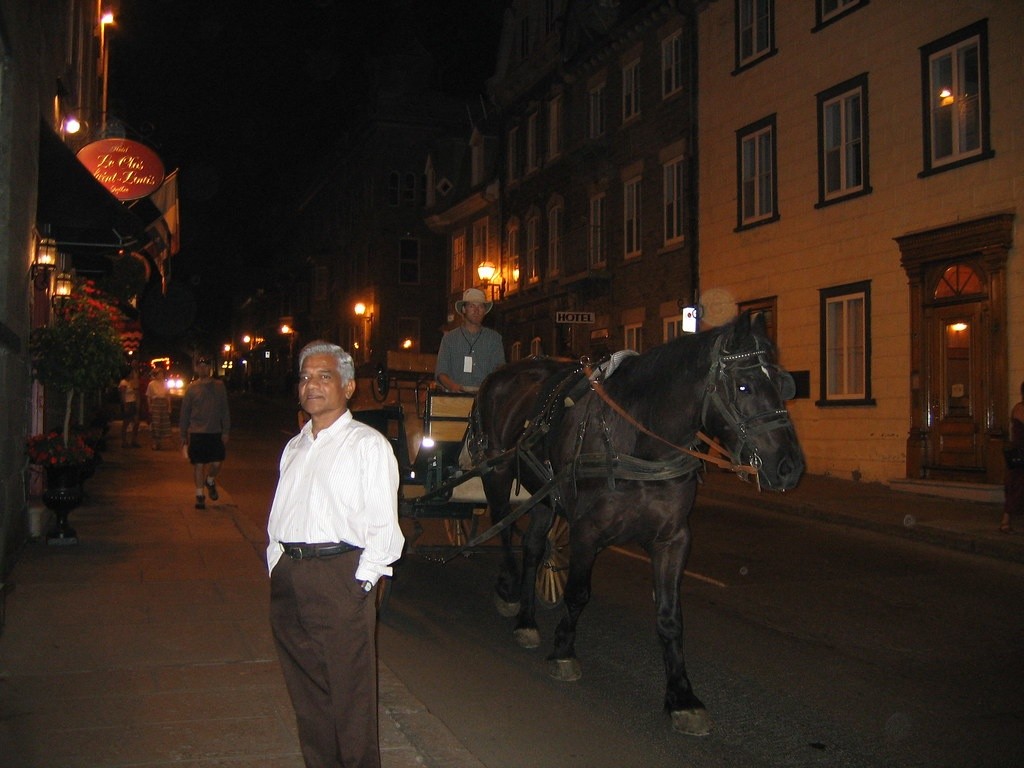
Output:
[1000,523,1017,535]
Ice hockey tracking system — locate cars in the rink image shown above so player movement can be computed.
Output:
[164,375,184,391]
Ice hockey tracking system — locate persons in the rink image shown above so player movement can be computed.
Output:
[434,286,506,456]
[180,354,231,510]
[997,382,1024,536]
[264,340,405,768]
[145,368,173,450]
[118,366,152,447]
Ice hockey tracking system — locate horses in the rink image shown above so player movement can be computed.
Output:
[467,307,805,735]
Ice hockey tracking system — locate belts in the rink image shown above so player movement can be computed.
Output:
[284,544,356,560]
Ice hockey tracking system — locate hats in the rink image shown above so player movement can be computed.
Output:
[454,288,493,316]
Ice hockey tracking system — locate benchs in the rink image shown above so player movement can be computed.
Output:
[367,349,438,466]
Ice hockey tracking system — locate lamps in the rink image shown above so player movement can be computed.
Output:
[354,303,373,322]
[60,110,81,134]
[477,261,506,300]
[31,234,57,291]
[677,289,704,333]
[51,273,73,317]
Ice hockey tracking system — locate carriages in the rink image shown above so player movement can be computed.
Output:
[298,309,807,738]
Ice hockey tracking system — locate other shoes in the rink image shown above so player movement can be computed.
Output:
[205,479,218,500]
[121,443,129,448]
[195,495,206,509]
[131,443,141,448]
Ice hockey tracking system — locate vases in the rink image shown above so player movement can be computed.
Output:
[85,457,95,477]
[42,463,79,539]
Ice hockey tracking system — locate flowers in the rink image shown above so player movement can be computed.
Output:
[27,433,94,463]
[80,430,102,447]
